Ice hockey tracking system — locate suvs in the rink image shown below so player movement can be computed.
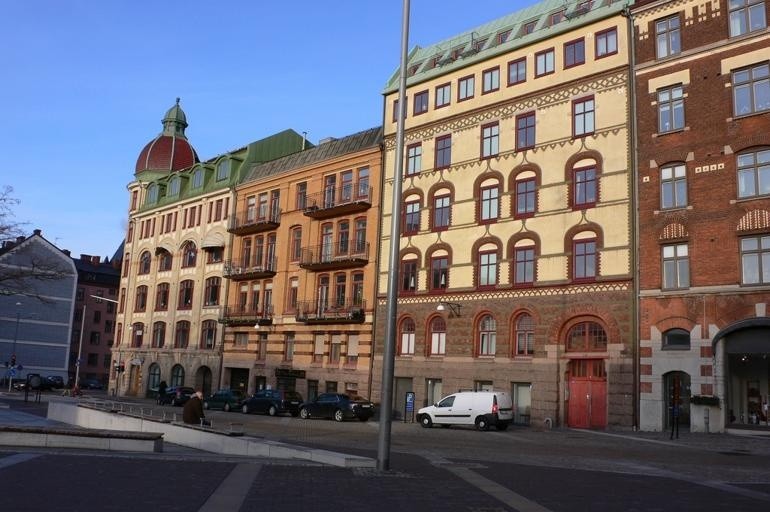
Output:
[242,390,304,417]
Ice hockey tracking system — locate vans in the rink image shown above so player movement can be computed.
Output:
[418,391,514,431]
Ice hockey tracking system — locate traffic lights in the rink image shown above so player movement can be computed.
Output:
[121,362,125,372]
[10,354,15,367]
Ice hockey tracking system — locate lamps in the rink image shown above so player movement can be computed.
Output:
[254,322,276,334]
[437,302,461,317]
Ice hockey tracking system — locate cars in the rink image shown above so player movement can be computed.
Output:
[79,379,98,390]
[298,393,375,422]
[203,389,247,411]
[14,376,63,393]
[158,386,195,406]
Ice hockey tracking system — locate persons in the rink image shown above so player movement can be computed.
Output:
[183,391,211,426]
[158,380,168,406]
